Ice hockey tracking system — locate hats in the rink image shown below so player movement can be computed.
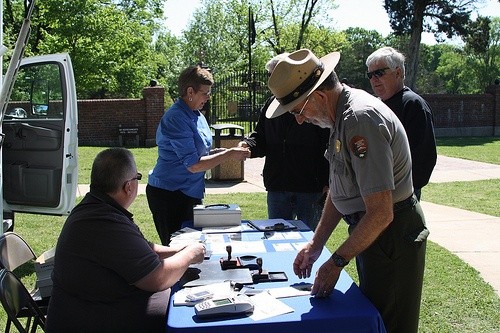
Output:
[265,49,341,119]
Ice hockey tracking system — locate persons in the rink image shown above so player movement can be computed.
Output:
[45,148,206,333]
[365,46,437,200]
[239,52,331,231]
[266,48,428,333]
[146,67,250,247]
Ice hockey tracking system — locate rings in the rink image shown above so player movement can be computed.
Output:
[203,245,205,248]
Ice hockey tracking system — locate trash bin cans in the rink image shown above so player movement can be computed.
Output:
[208,124,245,181]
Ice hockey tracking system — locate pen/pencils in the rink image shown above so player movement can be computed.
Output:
[247,223,254,229]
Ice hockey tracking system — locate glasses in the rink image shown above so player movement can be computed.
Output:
[268,72,272,77]
[128,172,142,182]
[289,93,314,117]
[367,67,398,79]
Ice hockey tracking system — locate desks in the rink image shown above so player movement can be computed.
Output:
[166,220,388,333]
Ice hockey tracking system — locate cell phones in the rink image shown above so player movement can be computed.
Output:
[186,291,213,301]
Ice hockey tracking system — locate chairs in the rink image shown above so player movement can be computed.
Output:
[0,269,46,333]
[0,231,50,333]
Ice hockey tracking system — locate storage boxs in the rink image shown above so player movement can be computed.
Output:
[33,246,56,298]
[193,204,242,227]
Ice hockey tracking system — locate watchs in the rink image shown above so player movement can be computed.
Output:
[332,252,349,267]
